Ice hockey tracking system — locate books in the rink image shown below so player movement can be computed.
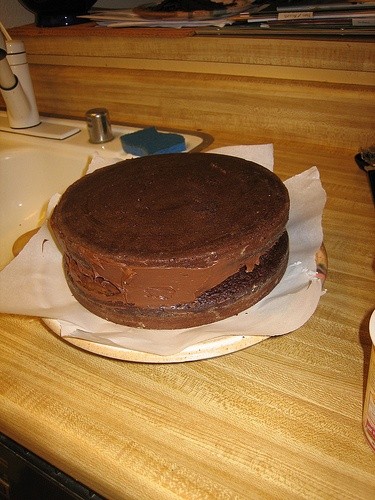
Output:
[70,1,375,33]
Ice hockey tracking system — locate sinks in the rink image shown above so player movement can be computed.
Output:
[0,109,213,272]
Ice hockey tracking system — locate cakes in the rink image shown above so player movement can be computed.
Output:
[48,153,291,328]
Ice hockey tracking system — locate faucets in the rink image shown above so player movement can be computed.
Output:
[0,40,40,128]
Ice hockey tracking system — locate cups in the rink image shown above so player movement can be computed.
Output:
[361,309,375,451]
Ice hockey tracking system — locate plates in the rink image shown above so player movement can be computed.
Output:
[39,243,329,365]
